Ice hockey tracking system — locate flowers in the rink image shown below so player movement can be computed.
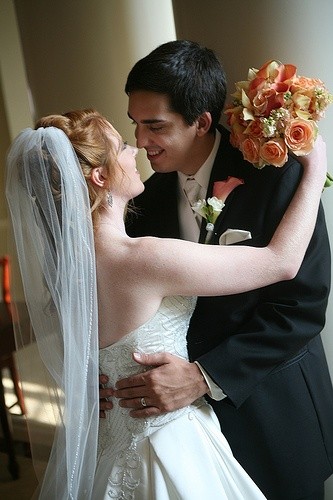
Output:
[191,176,245,231]
[224,58,333,192]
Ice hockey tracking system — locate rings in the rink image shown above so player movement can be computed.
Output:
[140,397,148,408]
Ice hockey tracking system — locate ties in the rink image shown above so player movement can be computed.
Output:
[183,180,203,228]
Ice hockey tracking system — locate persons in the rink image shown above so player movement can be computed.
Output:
[87,41,333,500]
[14,109,327,500]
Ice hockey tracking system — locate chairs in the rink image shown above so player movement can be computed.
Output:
[0,257,26,414]
